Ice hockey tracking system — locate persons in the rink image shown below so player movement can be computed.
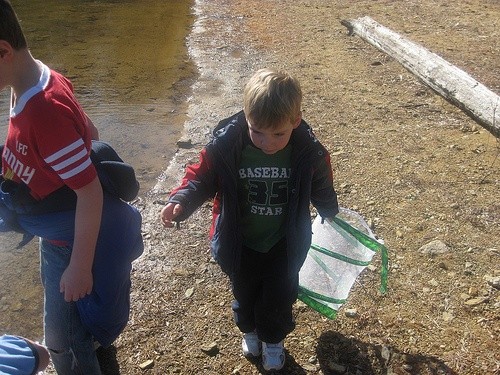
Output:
[0,0,144,375]
[161,67,339,373]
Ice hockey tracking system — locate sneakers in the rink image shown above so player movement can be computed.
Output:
[261,341,285,372]
[241,327,261,357]
